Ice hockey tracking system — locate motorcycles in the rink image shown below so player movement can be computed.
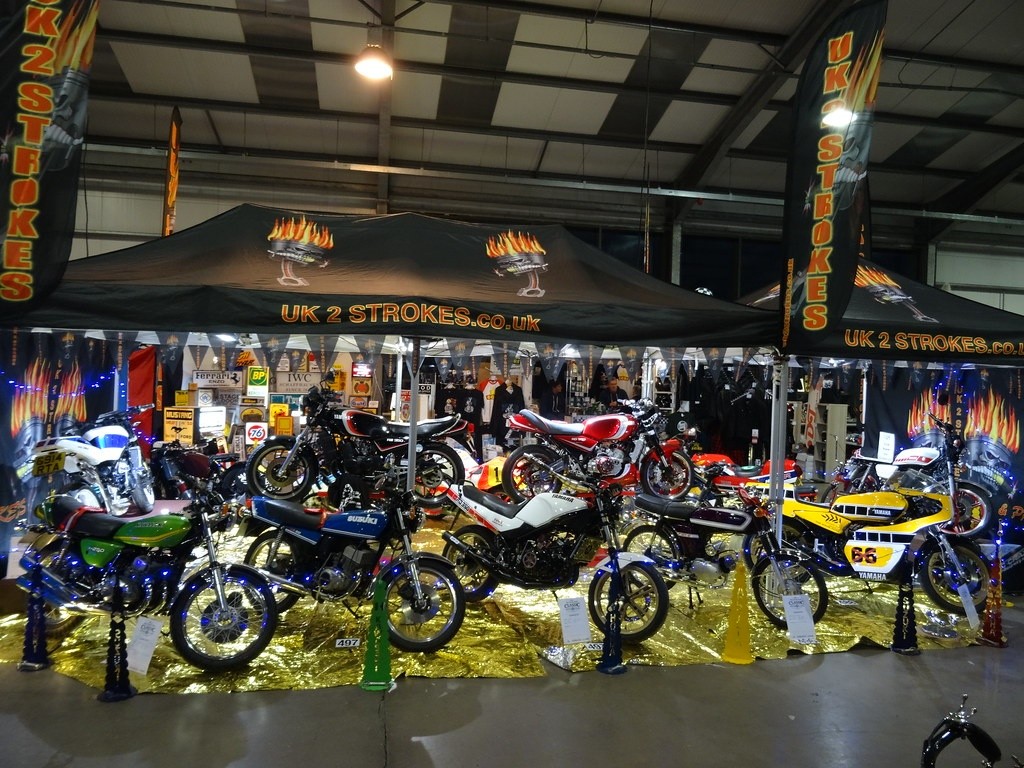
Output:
[15,403,1024,674]
[246,368,467,516]
[500,376,695,513]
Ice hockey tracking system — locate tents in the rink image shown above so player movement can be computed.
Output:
[1,204,791,608]
[732,254,1024,607]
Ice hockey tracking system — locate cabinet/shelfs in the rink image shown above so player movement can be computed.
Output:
[786,400,858,482]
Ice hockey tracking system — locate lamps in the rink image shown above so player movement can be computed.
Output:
[353,26,392,81]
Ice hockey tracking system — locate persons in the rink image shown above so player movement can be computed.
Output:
[434,382,462,445]
[461,383,484,462]
[478,375,504,426]
[490,379,525,452]
[599,377,629,413]
[538,381,565,421]
